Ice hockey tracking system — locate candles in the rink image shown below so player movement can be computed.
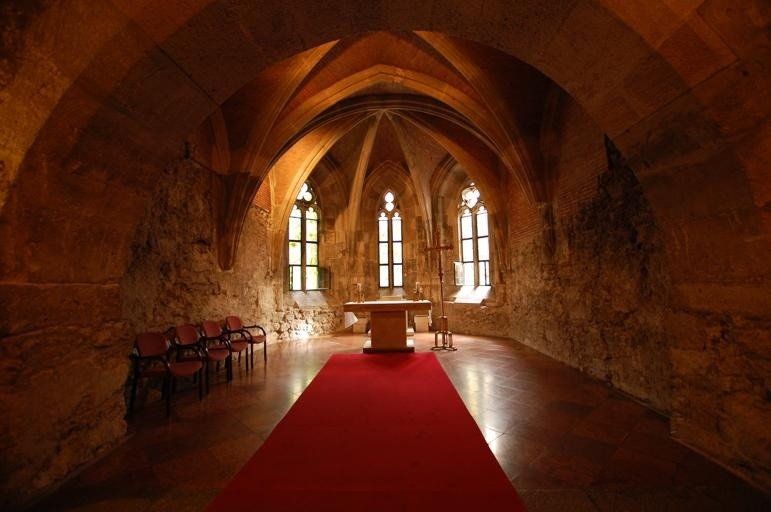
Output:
[416,282,420,293]
[357,283,361,294]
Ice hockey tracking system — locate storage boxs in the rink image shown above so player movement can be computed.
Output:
[353,317,368,334]
[414,315,429,332]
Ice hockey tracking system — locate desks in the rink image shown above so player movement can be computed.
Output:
[342,300,435,353]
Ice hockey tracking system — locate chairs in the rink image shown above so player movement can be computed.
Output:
[381,295,408,331]
[130,315,267,417]
